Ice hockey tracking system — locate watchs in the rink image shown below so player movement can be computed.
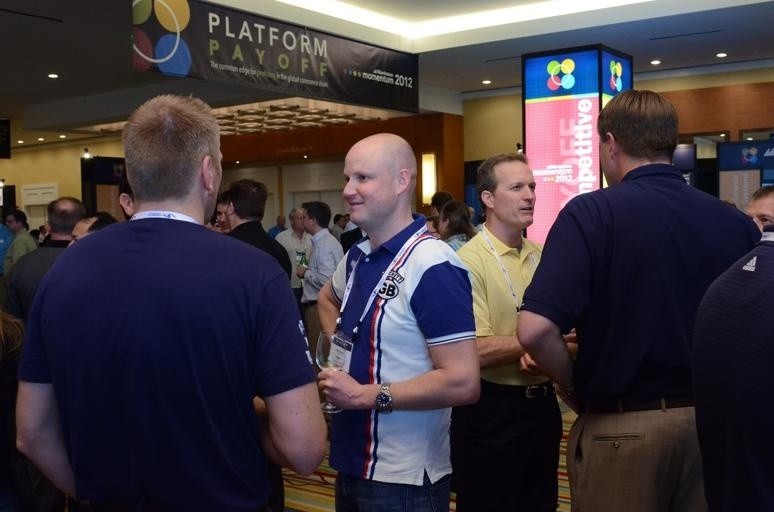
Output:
[377,382,392,411]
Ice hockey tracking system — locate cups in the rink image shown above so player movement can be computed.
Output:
[315,329,347,414]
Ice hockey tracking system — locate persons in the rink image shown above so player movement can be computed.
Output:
[16,97,329,511]
[68,212,118,250]
[0,205,15,273]
[269,215,289,239]
[333,213,357,232]
[690,225,773,511]
[438,199,475,252]
[318,132,482,512]
[431,191,453,216]
[222,180,293,282]
[469,208,477,232]
[426,217,443,242]
[275,208,313,304]
[0,277,26,512]
[517,88,761,512]
[4,210,39,277]
[450,153,563,512]
[28,230,40,242]
[7,197,86,318]
[340,225,368,255]
[296,202,345,362]
[214,189,233,234]
[40,225,47,243]
[743,186,774,234]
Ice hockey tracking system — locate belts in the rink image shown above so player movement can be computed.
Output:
[588,395,696,413]
[301,299,318,309]
[480,380,556,402]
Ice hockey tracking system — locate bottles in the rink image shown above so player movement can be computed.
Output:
[299,253,309,269]
